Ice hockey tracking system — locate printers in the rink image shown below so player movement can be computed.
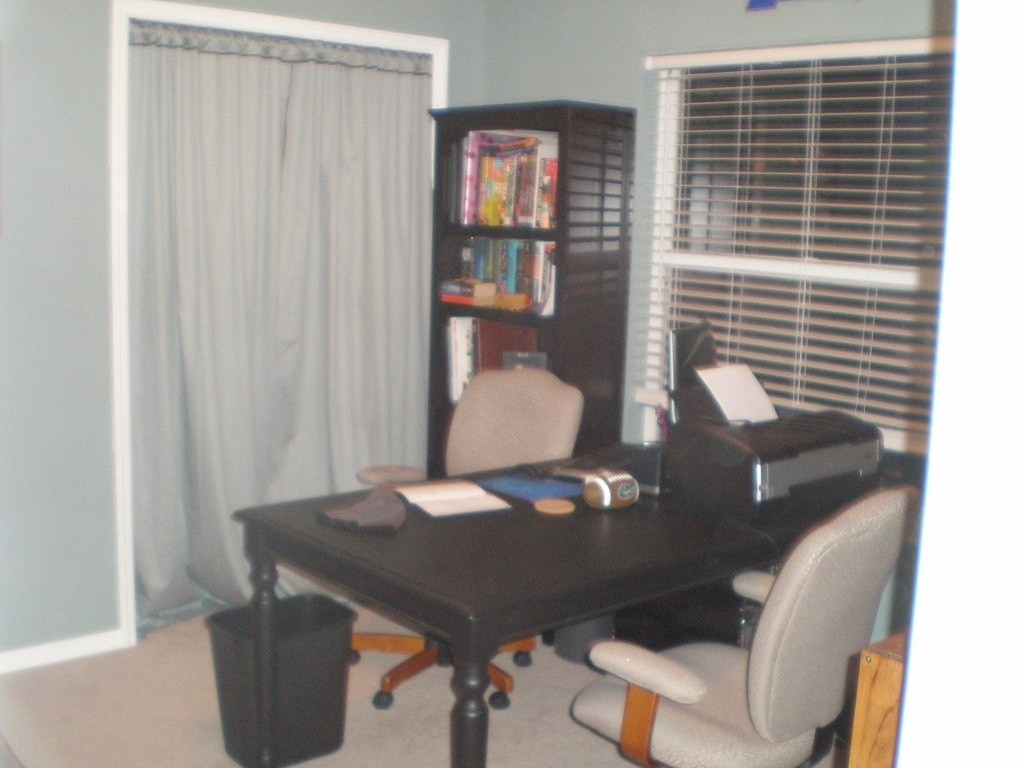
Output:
[662,382,884,523]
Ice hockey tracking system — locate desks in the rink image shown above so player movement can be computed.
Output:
[231,441,926,768]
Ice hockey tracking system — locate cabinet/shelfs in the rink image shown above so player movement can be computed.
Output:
[427,99,638,480]
[849,624,911,768]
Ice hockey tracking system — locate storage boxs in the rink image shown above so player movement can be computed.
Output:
[477,317,553,372]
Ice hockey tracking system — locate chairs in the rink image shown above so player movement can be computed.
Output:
[570,483,922,768]
[345,361,586,711]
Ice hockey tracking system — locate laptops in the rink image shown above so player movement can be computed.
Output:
[545,319,713,495]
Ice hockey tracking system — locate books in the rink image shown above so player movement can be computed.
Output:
[439,132,558,313]
[395,477,513,518]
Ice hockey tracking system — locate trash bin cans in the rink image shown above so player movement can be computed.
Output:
[202,591,355,768]
